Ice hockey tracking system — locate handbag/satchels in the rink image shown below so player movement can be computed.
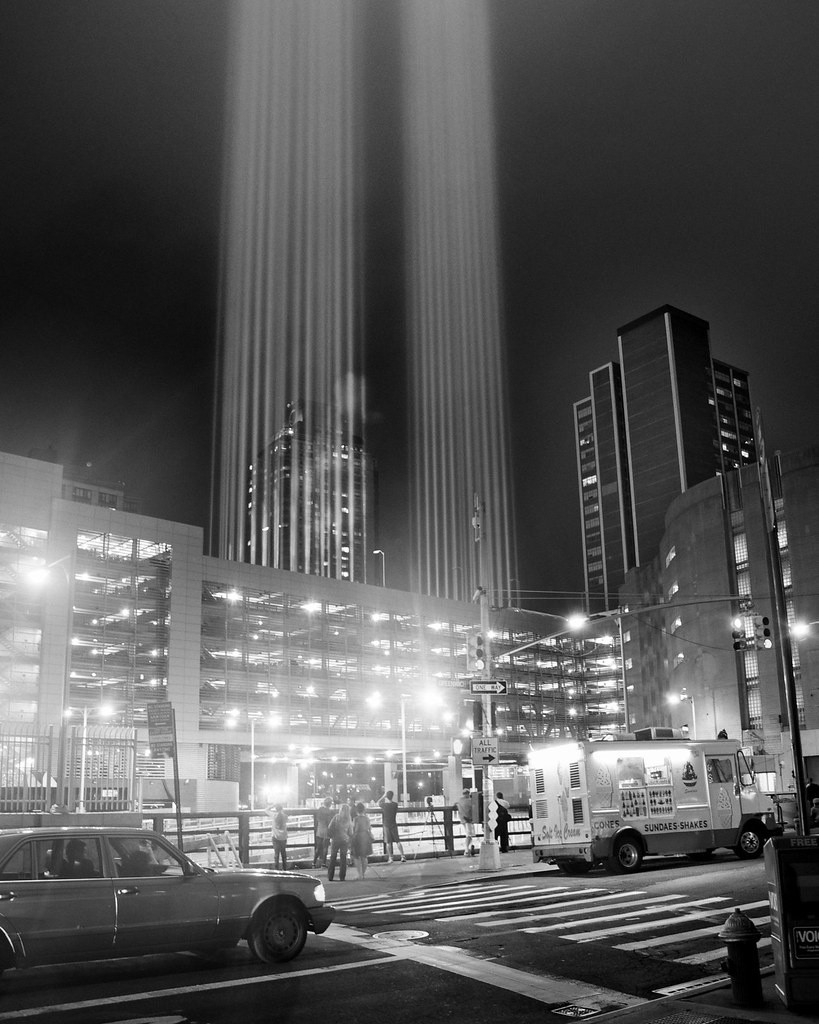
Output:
[368,832,375,843]
[497,807,511,823]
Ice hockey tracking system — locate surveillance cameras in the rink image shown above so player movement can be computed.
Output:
[472,517,481,529]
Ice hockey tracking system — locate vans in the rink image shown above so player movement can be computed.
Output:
[527,726,784,877]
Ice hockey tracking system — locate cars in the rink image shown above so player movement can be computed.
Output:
[0,829,336,977]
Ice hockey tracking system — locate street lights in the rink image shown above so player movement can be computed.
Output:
[366,688,444,832]
[63,704,112,811]
[568,605,630,735]
[224,715,283,810]
[373,550,386,587]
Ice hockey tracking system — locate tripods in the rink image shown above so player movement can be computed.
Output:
[413,806,453,860]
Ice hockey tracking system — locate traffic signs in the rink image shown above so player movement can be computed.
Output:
[472,737,500,765]
[470,679,508,695]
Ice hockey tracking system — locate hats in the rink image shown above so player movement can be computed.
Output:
[324,798,332,803]
[463,788,470,795]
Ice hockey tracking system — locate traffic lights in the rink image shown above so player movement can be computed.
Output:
[731,615,748,652]
[752,612,773,651]
[466,634,485,671]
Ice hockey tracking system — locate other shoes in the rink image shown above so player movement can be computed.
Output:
[340,877,345,881]
[388,857,393,864]
[312,863,316,868]
[320,864,327,868]
[464,849,471,856]
[329,877,333,881]
[352,875,366,881]
[400,855,406,862]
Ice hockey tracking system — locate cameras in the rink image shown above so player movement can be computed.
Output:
[427,798,432,803]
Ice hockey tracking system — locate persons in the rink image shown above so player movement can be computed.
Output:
[377,790,407,864]
[265,802,290,870]
[351,802,373,881]
[133,852,157,877]
[494,792,511,853]
[326,804,353,882]
[805,777,819,809]
[347,796,359,867]
[312,797,335,870]
[453,789,473,857]
[67,840,101,878]
